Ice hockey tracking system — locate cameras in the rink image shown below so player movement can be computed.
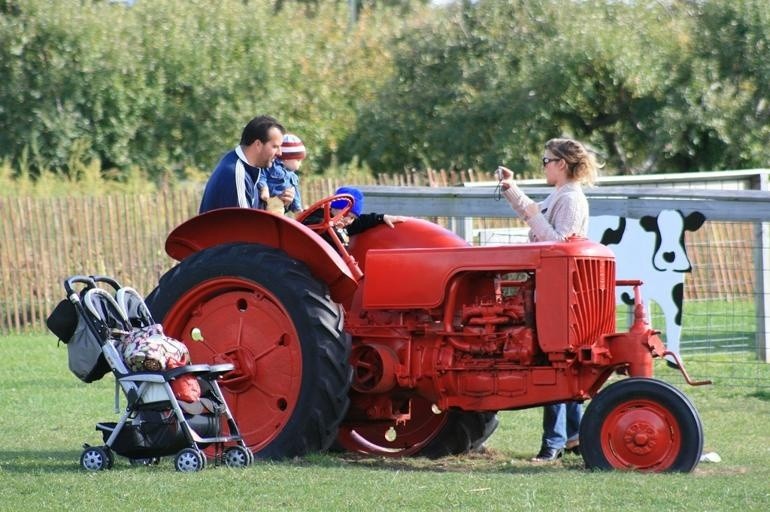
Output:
[495,168,505,182]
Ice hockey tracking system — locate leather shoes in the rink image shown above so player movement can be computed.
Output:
[565,442,581,454]
[535,446,564,460]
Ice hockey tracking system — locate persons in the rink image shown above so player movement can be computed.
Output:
[497,135,597,462]
[199,115,408,258]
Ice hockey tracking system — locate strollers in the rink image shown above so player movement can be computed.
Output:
[64,276,255,473]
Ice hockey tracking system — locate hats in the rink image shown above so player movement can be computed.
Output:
[276,134,306,160]
[329,187,365,217]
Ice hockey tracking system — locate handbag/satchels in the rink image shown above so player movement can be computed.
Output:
[121,323,190,371]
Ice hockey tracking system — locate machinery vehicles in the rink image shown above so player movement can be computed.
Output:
[143,194,703,472]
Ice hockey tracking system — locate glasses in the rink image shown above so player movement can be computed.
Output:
[542,158,561,168]
